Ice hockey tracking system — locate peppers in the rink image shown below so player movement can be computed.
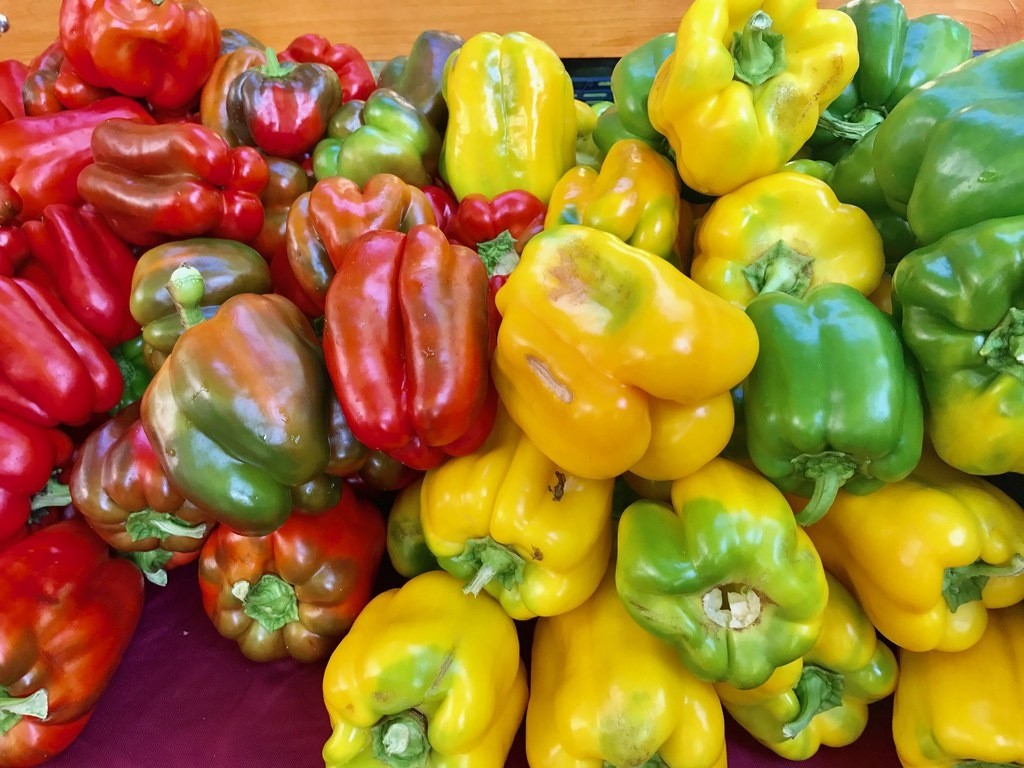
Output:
[0,1,1024,768]
